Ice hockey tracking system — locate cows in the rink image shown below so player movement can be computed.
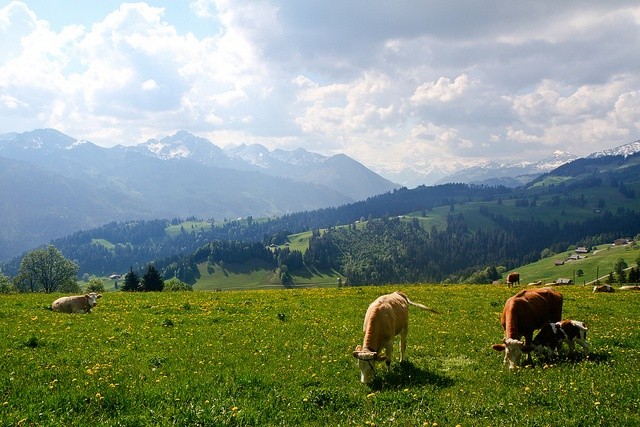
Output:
[506,272,520,289]
[493,288,563,372]
[351,290,441,385]
[532,319,589,359]
[593,285,616,293]
[52,292,102,315]
